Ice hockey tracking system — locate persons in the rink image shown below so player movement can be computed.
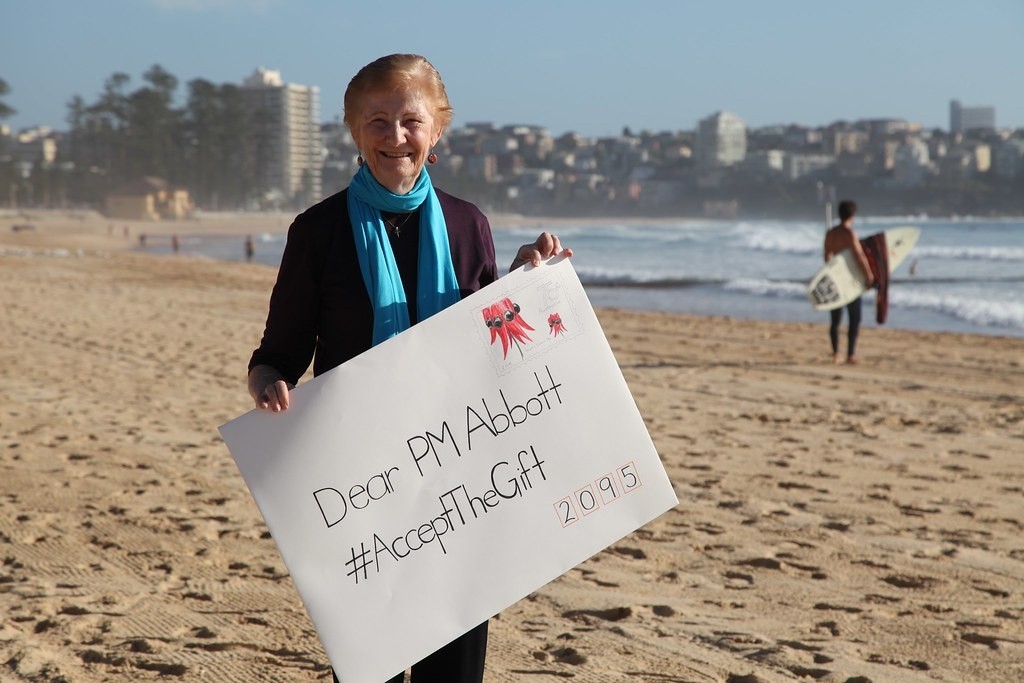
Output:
[246,235,254,261]
[139,234,146,246]
[823,200,875,366]
[171,233,178,254]
[245,52,572,683]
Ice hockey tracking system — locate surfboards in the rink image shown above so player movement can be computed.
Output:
[806,226,921,312]
